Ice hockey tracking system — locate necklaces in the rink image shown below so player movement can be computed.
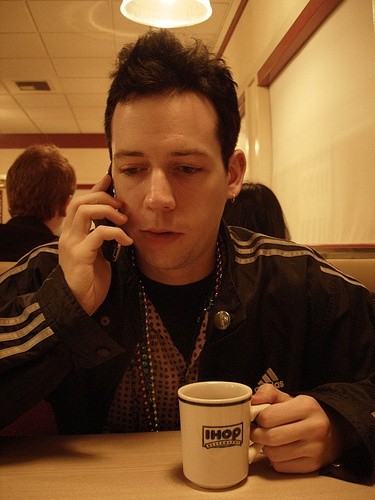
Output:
[128,239,224,433]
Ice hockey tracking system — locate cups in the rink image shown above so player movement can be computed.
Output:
[177,380,272,491]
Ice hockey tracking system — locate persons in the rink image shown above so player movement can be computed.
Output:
[0,144,77,261]
[221,182,286,239]
[0,26,375,484]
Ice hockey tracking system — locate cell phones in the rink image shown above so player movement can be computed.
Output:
[94,162,122,262]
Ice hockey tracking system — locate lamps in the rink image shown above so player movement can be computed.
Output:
[119,0,213,29]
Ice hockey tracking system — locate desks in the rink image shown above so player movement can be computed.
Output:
[0,427,374,500]
